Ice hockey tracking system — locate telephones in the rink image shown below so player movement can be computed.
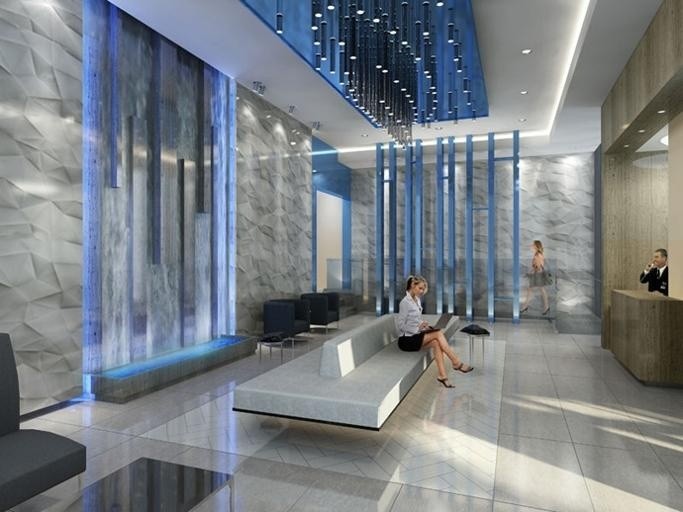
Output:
[648,260,655,268]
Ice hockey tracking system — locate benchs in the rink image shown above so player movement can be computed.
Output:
[231,312,463,433]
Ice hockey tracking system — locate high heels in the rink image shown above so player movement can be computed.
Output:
[437,376,456,388]
[453,362,474,374]
[542,307,551,316]
[520,306,529,314]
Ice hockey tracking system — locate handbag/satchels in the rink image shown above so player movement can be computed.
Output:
[526,263,554,288]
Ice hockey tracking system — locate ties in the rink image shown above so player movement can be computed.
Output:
[657,269,660,279]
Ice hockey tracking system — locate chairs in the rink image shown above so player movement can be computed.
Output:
[300,291,341,336]
[0,329,89,512]
[262,298,314,345]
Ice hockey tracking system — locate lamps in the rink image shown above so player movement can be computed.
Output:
[247,80,324,133]
